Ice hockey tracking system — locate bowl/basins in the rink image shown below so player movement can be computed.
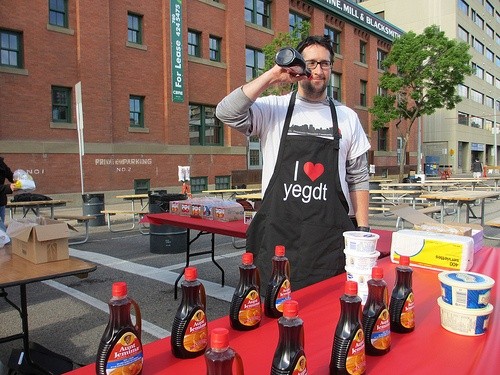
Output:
[343,230,380,255]
[343,250,381,269]
[437,296,494,336]
[344,265,372,306]
[438,270,496,310]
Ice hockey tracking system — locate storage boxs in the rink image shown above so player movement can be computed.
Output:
[389,204,484,252]
[391,229,474,273]
[7,217,79,264]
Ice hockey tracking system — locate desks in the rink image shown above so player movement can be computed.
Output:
[138,213,392,299]
[368,177,500,239]
[231,193,261,249]
[201,188,261,201]
[6,199,89,246]
[107,194,150,236]
[62,246,500,375]
[0,245,97,375]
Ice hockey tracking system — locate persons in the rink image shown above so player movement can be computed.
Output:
[0,153,20,232]
[471,158,482,184]
[215,35,371,300]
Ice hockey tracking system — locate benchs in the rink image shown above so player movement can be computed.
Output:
[484,216,500,241]
[370,202,433,207]
[43,214,96,220]
[414,206,449,214]
[371,196,426,200]
[100,209,146,214]
[368,207,390,211]
[451,186,500,191]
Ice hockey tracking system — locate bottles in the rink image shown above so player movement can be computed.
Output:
[275,46,313,80]
[228,252,262,331]
[264,245,292,318]
[328,280,367,375]
[270,300,308,375]
[204,327,244,375]
[95,281,144,375]
[362,267,392,357]
[170,266,209,360]
[389,255,416,335]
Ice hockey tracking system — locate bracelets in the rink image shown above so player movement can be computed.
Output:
[357,226,371,232]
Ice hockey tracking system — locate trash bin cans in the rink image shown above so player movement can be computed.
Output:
[149,193,187,254]
[148,190,167,196]
[232,185,246,201]
[426,163,439,176]
[404,177,422,206]
[368,182,381,214]
[82,193,105,227]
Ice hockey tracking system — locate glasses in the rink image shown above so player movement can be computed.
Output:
[305,60,333,69]
